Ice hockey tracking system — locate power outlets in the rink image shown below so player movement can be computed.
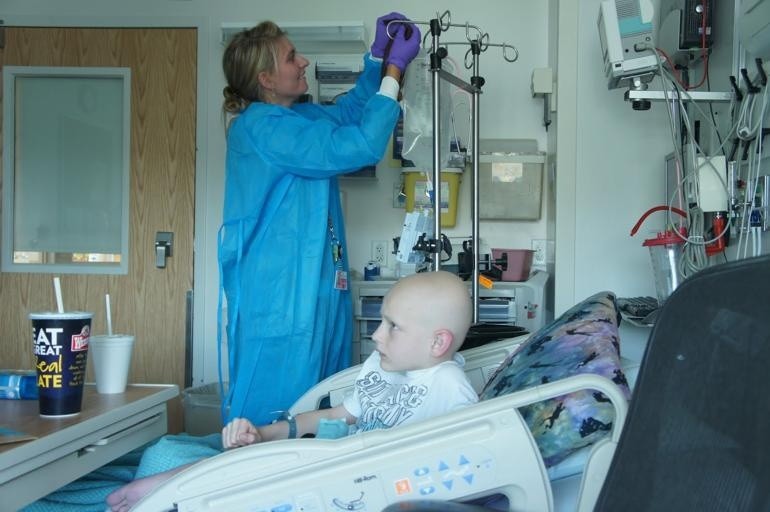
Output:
[371,241,388,267]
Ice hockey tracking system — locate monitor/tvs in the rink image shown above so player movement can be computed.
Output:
[663,145,690,229]
[596,0,658,89]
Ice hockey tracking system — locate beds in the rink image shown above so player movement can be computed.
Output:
[22,330,641,512]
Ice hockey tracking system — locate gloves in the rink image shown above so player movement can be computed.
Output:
[382,18,423,77]
[369,12,406,60]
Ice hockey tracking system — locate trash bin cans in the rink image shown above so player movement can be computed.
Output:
[182,382,229,434]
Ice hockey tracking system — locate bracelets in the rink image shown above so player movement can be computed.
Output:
[269,412,297,441]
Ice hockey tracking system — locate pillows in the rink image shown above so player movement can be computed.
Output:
[476,290,634,470]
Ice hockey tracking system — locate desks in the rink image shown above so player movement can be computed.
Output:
[1,381,180,511]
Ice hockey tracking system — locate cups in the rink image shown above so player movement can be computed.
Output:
[88,335,136,394]
[27,311,94,419]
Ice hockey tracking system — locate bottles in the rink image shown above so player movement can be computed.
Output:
[0,373,40,400]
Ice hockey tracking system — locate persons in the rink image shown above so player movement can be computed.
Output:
[105,271,474,512]
[215,12,422,429]
